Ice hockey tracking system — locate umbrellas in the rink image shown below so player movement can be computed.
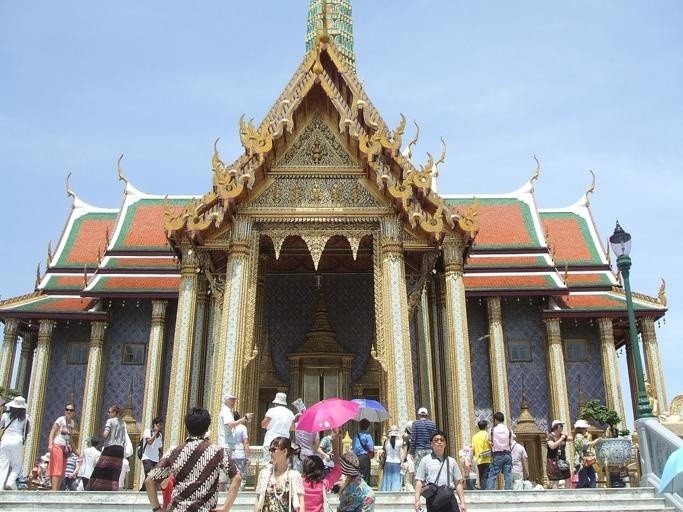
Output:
[657,447,683,495]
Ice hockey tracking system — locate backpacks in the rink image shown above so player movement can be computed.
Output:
[137,438,148,460]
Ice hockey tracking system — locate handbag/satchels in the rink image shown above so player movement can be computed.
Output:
[124,434,133,458]
[378,450,386,469]
[63,444,72,458]
[421,483,437,499]
[582,457,596,467]
[426,484,452,511]
[366,450,374,459]
[478,450,492,458]
[320,485,334,511]
[556,460,570,470]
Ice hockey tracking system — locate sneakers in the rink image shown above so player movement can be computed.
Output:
[4,485,12,490]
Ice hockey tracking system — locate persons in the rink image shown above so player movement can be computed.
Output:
[544,419,604,489]
[381,407,530,511]
[0,393,164,491]
[145,393,376,512]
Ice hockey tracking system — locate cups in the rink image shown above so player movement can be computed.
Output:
[246,412,254,422]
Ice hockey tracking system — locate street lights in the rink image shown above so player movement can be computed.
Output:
[609,221,658,422]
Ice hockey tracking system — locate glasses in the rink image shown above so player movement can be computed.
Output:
[434,438,445,442]
[269,447,281,452]
[559,426,563,427]
[65,408,74,412]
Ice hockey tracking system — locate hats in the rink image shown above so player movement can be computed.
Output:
[551,420,565,428]
[574,420,590,429]
[407,420,413,433]
[5,396,27,408]
[387,430,399,436]
[272,392,287,405]
[340,452,364,476]
[224,395,237,400]
[417,407,428,415]
